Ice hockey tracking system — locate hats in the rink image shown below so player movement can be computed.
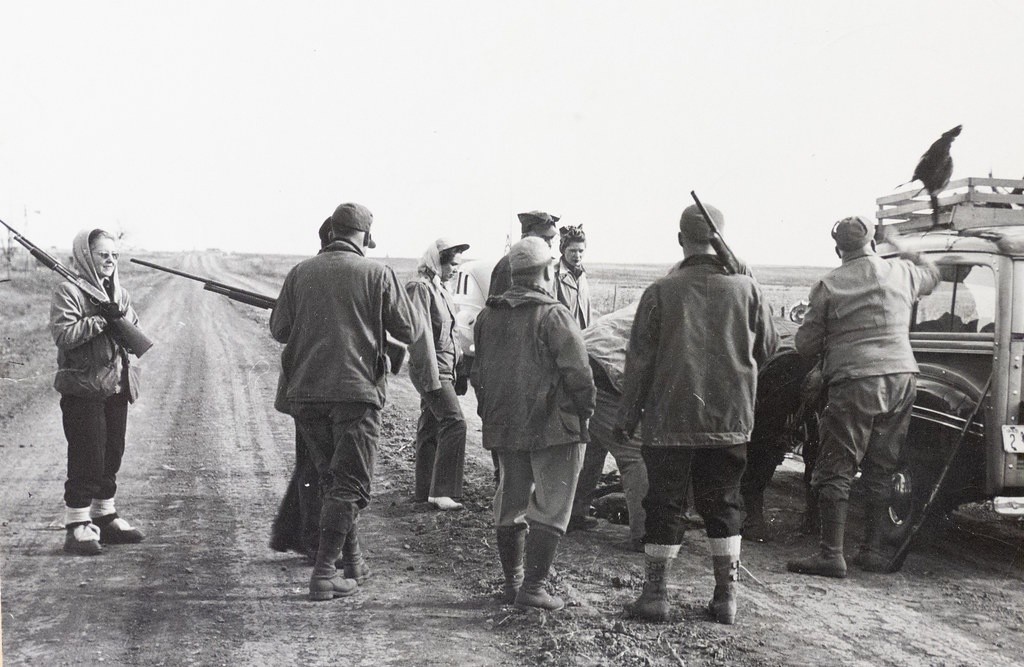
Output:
[680,202,725,238]
[333,203,377,248]
[518,210,560,237]
[319,216,332,239]
[509,236,562,270]
[436,237,470,252]
[831,216,875,248]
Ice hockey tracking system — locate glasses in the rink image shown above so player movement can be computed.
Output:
[91,251,120,261]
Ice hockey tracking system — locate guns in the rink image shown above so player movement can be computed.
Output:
[690,188,740,276]
[889,372,996,573]
[0,218,153,361]
[130,255,407,375]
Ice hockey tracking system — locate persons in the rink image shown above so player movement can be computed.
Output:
[552,223,593,330]
[567,298,650,552]
[406,236,471,510]
[786,215,941,578]
[49,227,147,555]
[740,313,829,536]
[613,204,780,621]
[489,210,561,485]
[268,202,425,599]
[269,213,392,569]
[471,235,598,611]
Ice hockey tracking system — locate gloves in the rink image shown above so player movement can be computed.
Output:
[454,377,468,396]
[613,423,635,444]
[100,302,125,322]
[429,389,441,397]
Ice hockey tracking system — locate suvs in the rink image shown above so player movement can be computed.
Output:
[872,171,1023,558]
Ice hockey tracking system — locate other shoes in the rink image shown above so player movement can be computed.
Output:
[566,516,598,532]
[428,496,464,511]
[63,521,102,556]
[92,513,143,544]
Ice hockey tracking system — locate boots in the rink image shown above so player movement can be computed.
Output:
[309,499,358,600]
[787,500,847,577]
[852,508,887,571]
[342,504,371,585]
[708,534,742,624]
[515,525,565,612]
[624,542,681,621]
[497,526,555,599]
